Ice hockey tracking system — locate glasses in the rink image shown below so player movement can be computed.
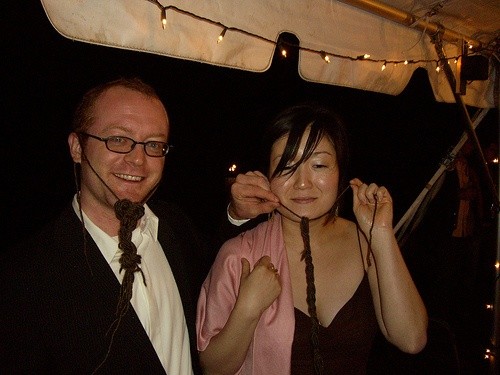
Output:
[76,131,175,157]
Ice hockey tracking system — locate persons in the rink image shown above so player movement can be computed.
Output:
[195,103,429,375]
[448,134,500,241]
[0,73,281,375]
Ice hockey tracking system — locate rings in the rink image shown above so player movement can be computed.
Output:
[267,265,279,275]
